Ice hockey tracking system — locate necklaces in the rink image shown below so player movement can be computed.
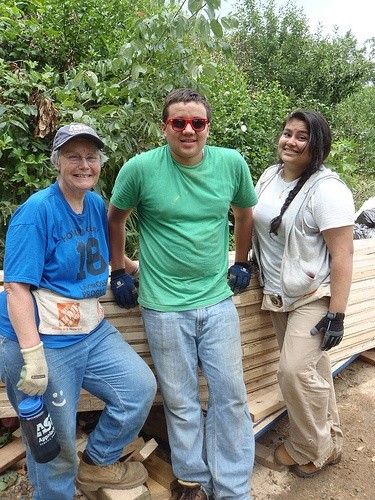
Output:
[278,184,291,197]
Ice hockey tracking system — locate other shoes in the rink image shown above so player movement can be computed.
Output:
[275,441,297,466]
[294,449,341,479]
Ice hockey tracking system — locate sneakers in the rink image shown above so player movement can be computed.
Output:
[169,478,212,500]
[76,456,148,492]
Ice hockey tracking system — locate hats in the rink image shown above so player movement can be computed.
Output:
[53,122,104,152]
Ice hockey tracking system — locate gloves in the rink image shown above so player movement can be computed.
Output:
[109,267,139,309]
[227,261,253,292]
[130,266,141,281]
[16,341,48,396]
[310,312,346,351]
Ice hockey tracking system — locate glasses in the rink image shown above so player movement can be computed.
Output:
[166,117,209,132]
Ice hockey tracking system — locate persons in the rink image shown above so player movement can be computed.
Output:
[250,110,355,477]
[107,88,258,500]
[0,123,157,500]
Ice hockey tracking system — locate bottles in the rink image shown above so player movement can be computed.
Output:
[17,394,61,464]
